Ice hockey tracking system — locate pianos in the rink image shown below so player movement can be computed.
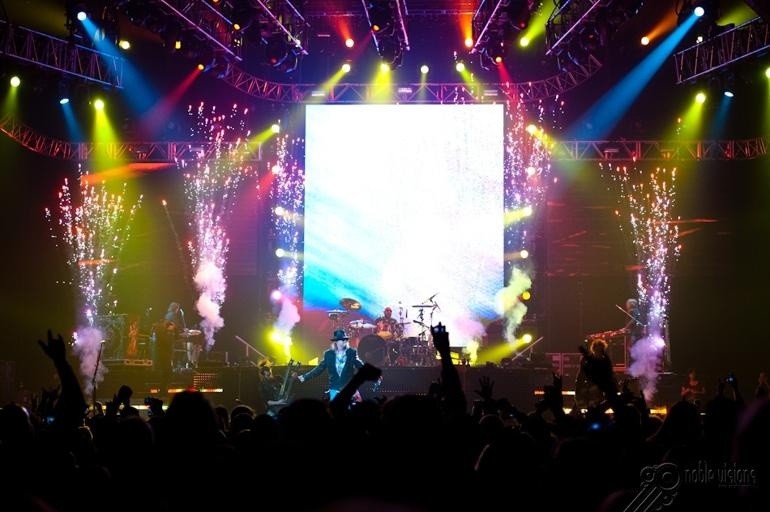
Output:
[586,328,634,341]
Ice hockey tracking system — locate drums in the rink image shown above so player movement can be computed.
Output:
[379,331,393,343]
[357,333,393,368]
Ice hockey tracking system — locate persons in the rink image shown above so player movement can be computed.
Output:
[1,322,770,510]
[623,298,641,369]
[165,302,192,369]
[374,308,403,337]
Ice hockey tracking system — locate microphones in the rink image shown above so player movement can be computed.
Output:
[413,319,424,326]
[100,339,106,344]
[180,309,184,316]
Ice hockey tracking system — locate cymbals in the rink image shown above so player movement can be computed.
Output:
[339,299,361,310]
[327,310,351,313]
[412,305,432,307]
[393,322,411,327]
[350,320,377,328]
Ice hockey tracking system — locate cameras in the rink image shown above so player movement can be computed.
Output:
[433,326,446,333]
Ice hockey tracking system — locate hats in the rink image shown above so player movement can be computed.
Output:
[329,329,350,341]
